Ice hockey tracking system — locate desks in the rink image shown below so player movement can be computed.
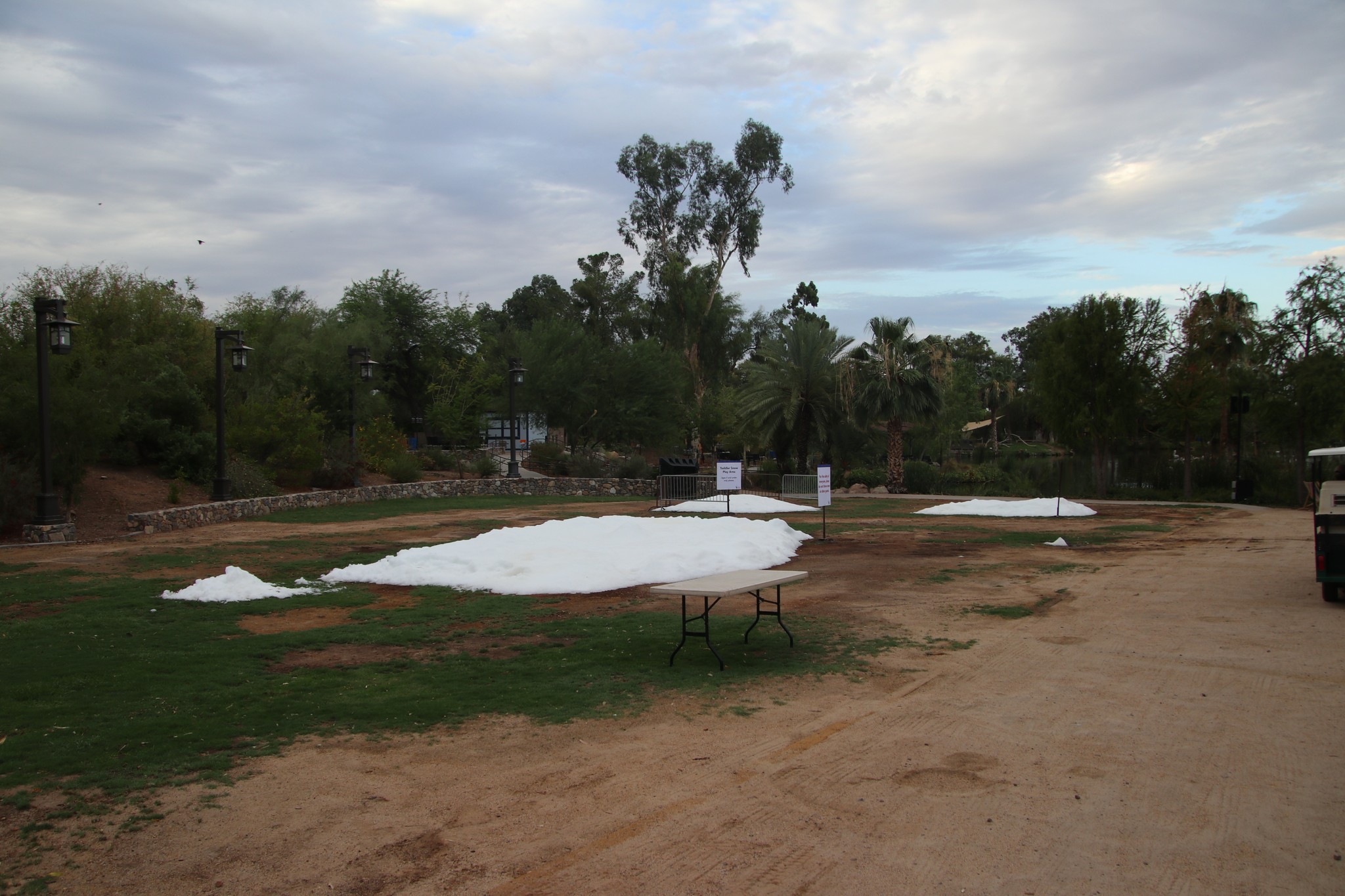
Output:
[649,569,809,672]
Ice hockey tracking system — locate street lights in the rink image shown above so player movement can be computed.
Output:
[345,344,379,468]
[214,325,255,499]
[506,356,530,479]
[30,296,81,524]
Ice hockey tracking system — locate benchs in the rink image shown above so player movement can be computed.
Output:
[747,454,761,465]
[1314,480,1345,535]
